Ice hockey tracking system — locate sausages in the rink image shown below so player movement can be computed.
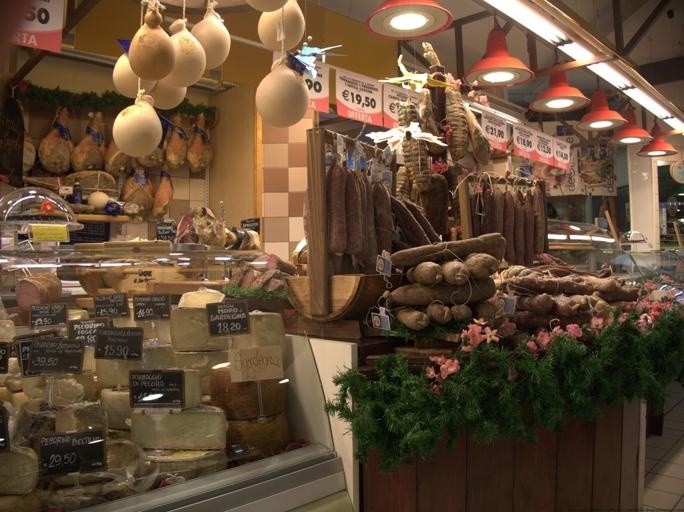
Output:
[326,162,639,332]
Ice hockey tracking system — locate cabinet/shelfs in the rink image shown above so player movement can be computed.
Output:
[546,219,684,292]
[0,249,354,512]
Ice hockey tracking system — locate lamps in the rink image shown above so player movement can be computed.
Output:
[462,15,531,88]
[478,2,683,133]
[610,99,652,145]
[639,115,678,157]
[366,0,450,39]
[529,51,590,112]
[578,81,627,127]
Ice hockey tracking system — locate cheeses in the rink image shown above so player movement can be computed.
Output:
[0,267,290,512]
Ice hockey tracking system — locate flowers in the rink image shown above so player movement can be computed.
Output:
[324,272,684,476]
